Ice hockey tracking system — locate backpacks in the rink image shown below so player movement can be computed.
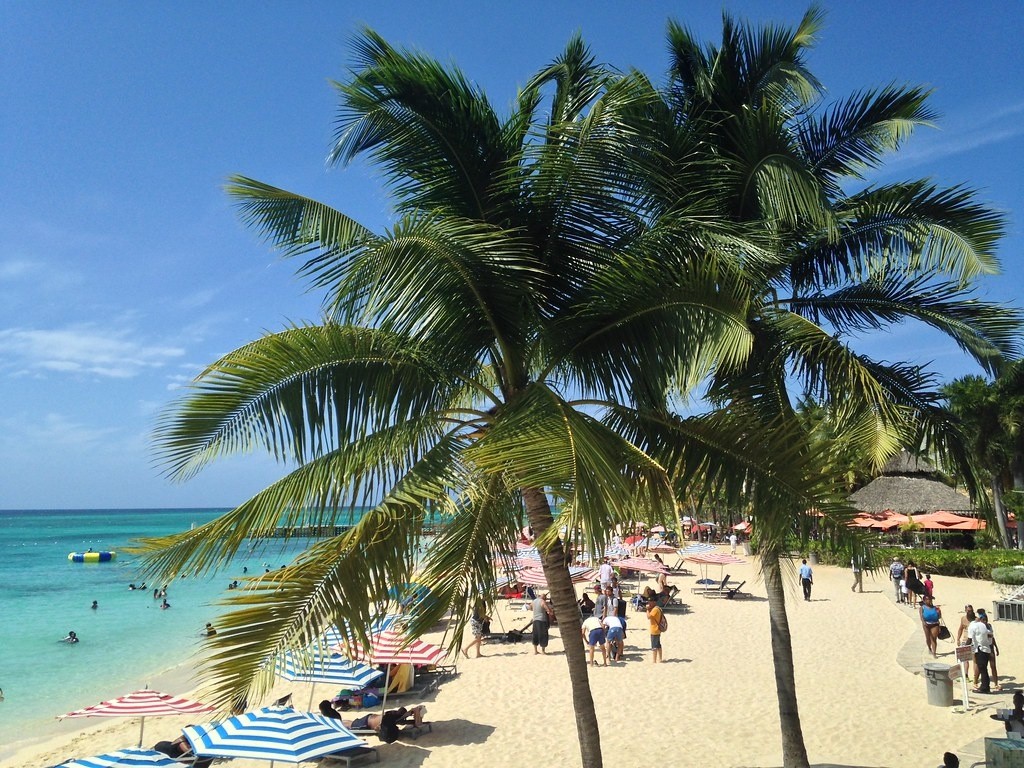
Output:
[604,596,626,618]
[652,606,667,632]
[891,564,903,579]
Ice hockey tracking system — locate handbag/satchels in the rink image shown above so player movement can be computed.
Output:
[362,693,381,708]
[937,617,951,640]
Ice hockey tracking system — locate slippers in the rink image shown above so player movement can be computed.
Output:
[477,654,484,658]
[462,649,469,659]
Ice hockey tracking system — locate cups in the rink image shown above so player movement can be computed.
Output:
[996,708,1009,719]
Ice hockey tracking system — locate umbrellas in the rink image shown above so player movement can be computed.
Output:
[47,613,448,768]
[839,450,983,514]
[418,516,751,599]
[744,525,752,534]
[798,508,1018,550]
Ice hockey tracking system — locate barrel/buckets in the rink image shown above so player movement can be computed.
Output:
[743,542,753,556]
[809,551,819,564]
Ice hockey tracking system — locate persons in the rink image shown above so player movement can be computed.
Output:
[799,560,814,602]
[340,707,407,730]
[972,609,1004,690]
[168,725,196,763]
[965,613,994,694]
[850,555,865,593]
[318,698,342,724]
[888,557,934,608]
[919,595,942,659]
[63,564,286,644]
[730,533,737,555]
[956,604,978,683]
[673,534,679,548]
[461,554,679,666]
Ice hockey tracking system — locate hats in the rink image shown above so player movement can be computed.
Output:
[646,596,658,601]
[594,585,601,590]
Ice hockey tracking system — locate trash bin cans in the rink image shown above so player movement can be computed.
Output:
[923,662,953,707]
[742,542,753,555]
[808,550,819,564]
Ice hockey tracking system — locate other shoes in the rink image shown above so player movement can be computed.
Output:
[906,602,908,605]
[956,677,969,683]
[859,589,863,593]
[909,604,912,607]
[913,606,916,609]
[851,586,855,592]
[973,684,979,689]
[972,688,990,694]
[901,599,904,602]
[896,600,900,603]
[994,684,1000,688]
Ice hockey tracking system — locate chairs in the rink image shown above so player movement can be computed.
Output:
[150,664,458,768]
[482,558,752,645]
